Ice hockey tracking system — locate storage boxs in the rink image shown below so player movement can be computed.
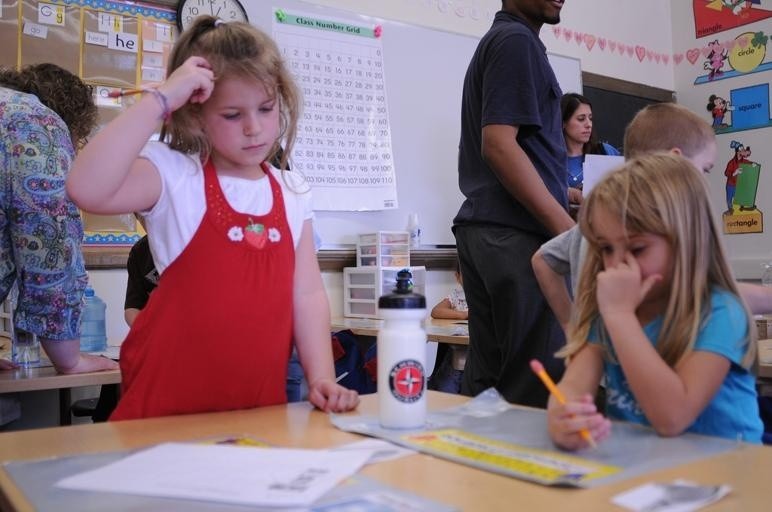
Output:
[344,267,425,319]
[355,230,411,267]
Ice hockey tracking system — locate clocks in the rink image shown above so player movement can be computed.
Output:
[177,0,249,36]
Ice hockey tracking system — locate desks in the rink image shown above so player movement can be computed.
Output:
[0,347,121,429]
[1,390,772,512]
[325,317,470,346]
[757,338,771,380]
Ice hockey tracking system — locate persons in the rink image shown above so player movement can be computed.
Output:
[561,92,623,204]
[451,0,583,410]
[546,152,764,452]
[66,16,361,422]
[1,62,119,376]
[92,213,159,424]
[532,103,719,419]
[431,263,468,395]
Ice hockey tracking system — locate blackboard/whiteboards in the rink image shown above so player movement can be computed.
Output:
[250,2,583,255]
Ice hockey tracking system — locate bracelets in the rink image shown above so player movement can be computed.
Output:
[142,88,172,127]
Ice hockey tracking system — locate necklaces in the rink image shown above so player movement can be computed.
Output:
[566,167,582,182]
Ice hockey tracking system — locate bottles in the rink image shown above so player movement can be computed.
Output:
[409,213,421,248]
[377,271,427,429]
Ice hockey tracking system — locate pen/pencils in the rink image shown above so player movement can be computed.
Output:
[530,360,599,451]
[107,83,161,99]
